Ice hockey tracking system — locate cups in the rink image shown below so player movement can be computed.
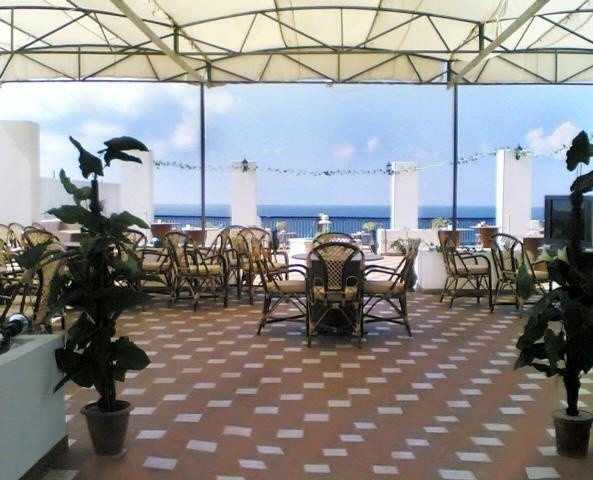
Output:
[185,223,190,229]
[157,218,161,224]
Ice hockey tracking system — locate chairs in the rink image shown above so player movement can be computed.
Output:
[305,232,365,349]
[249,238,312,336]
[361,238,420,337]
[438,230,491,309]
[121,228,288,311]
[490,232,552,320]
[0,223,67,334]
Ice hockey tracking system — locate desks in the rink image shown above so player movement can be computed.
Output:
[291,251,384,337]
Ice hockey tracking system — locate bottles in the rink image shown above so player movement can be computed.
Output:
[474,234,484,251]
[3,311,34,338]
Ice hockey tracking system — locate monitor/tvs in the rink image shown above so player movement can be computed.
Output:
[544,195,593,249]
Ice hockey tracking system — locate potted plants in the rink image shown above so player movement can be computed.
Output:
[513,128,593,456]
[389,235,419,292]
[45,135,153,456]
